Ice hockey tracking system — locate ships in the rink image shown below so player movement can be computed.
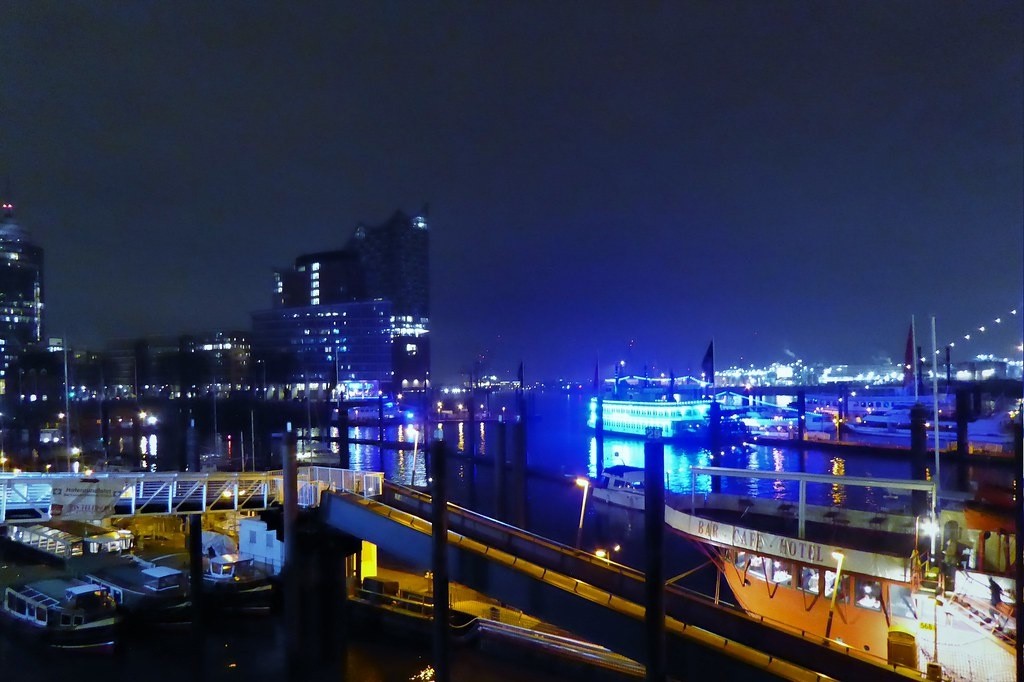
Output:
[588,376,722,440]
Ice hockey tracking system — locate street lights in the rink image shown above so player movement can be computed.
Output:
[575,478,591,552]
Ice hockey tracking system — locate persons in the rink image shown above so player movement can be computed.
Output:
[860,586,881,609]
[750,554,835,594]
[988,577,1004,616]
[969,532,991,569]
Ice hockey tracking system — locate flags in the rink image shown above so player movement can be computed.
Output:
[701,341,714,384]
[594,363,598,391]
[902,323,914,385]
[517,362,524,389]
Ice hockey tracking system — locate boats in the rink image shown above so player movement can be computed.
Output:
[0,575,125,653]
[202,547,273,602]
[665,315,1018,682]
[79,553,193,624]
[592,465,650,510]
